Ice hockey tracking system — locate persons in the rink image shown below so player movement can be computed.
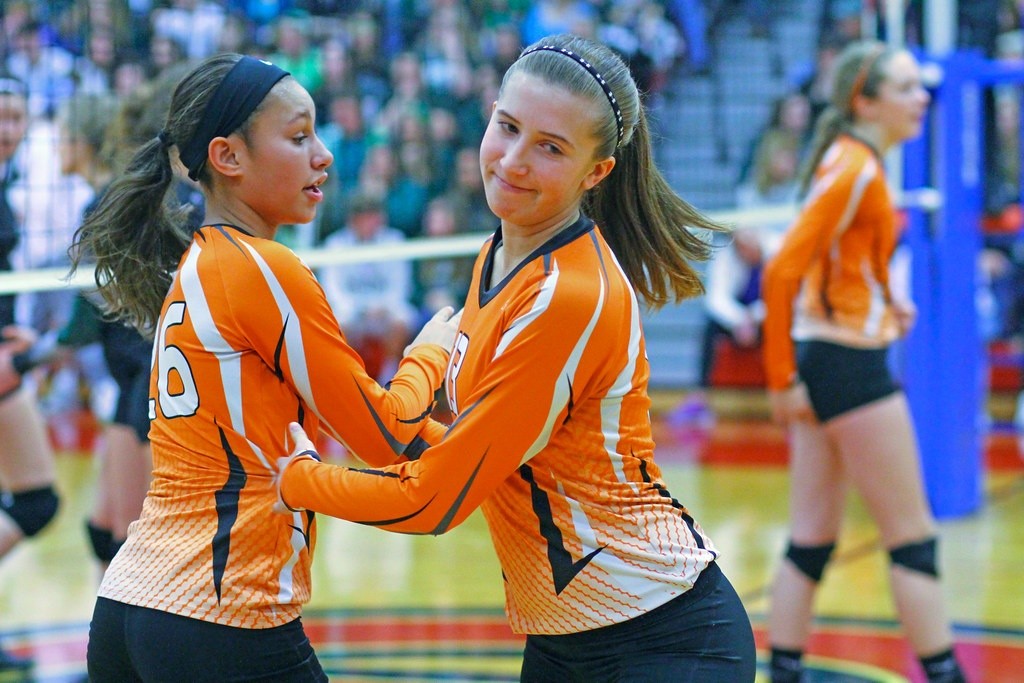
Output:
[762,39,966,683]
[56,51,466,683]
[272,34,756,683]
[0,0,1024,683]
